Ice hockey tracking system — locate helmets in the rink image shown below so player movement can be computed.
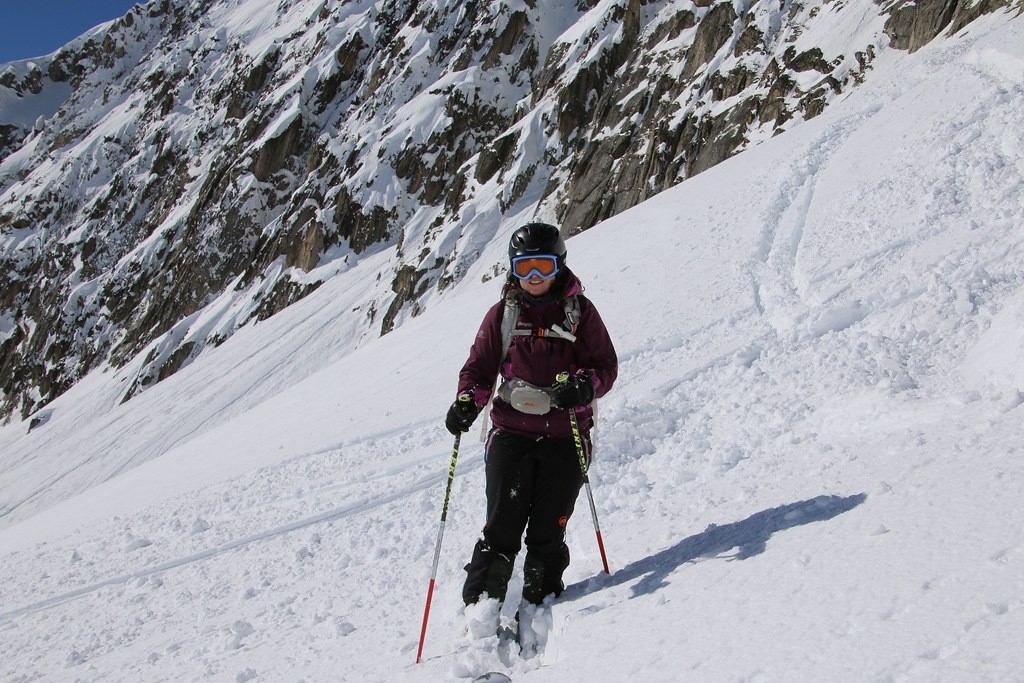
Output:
[508,223,567,268]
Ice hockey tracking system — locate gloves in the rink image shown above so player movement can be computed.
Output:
[445,399,477,434]
[551,375,594,409]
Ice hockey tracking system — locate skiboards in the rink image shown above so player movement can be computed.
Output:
[469,599,545,683]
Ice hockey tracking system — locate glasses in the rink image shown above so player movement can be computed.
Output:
[511,256,561,279]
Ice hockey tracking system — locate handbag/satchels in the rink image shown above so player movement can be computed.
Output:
[499,378,552,415]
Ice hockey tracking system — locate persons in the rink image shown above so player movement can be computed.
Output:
[446,224,618,658]
